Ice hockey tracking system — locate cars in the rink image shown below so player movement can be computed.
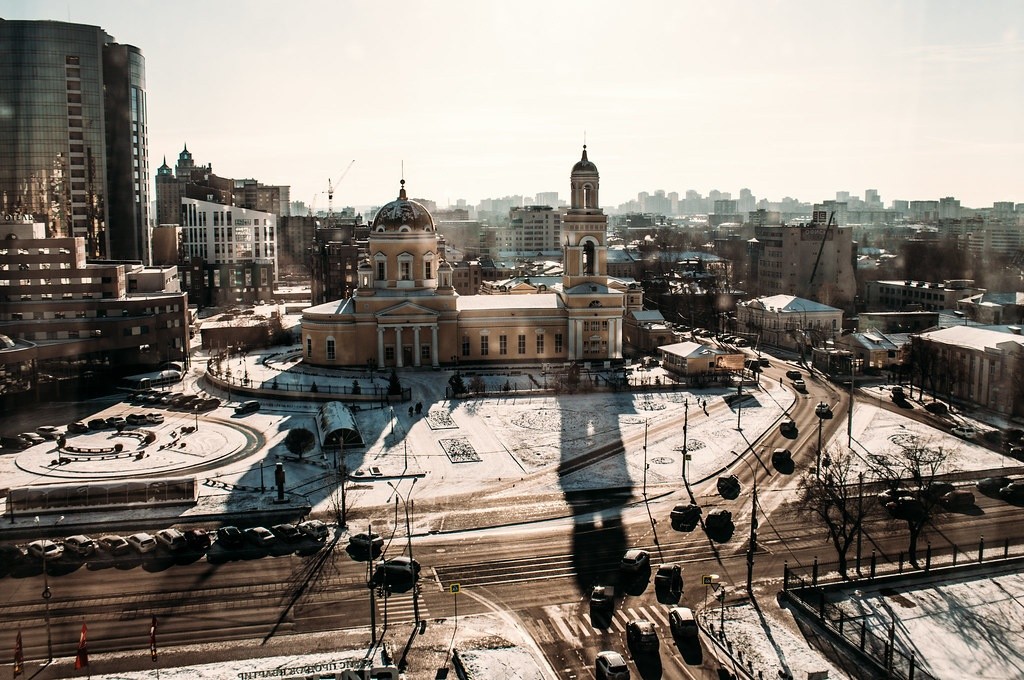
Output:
[26,539,63,562]
[705,508,733,529]
[717,474,739,490]
[816,403,831,415]
[219,298,286,321]
[589,584,616,611]
[99,534,130,557]
[1,389,221,459]
[655,563,681,585]
[784,368,806,390]
[771,419,797,465]
[349,532,383,549]
[880,384,1024,513]
[671,322,771,369]
[234,400,260,416]
[0,542,25,565]
[670,504,701,522]
[214,518,331,553]
[620,549,650,570]
[126,527,212,552]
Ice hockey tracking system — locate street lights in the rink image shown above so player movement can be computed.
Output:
[33,515,65,663]
[387,476,420,623]
[730,449,766,591]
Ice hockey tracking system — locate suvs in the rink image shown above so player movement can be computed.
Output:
[627,619,659,653]
[374,555,421,577]
[62,533,96,556]
[668,606,698,638]
[596,651,630,680]
